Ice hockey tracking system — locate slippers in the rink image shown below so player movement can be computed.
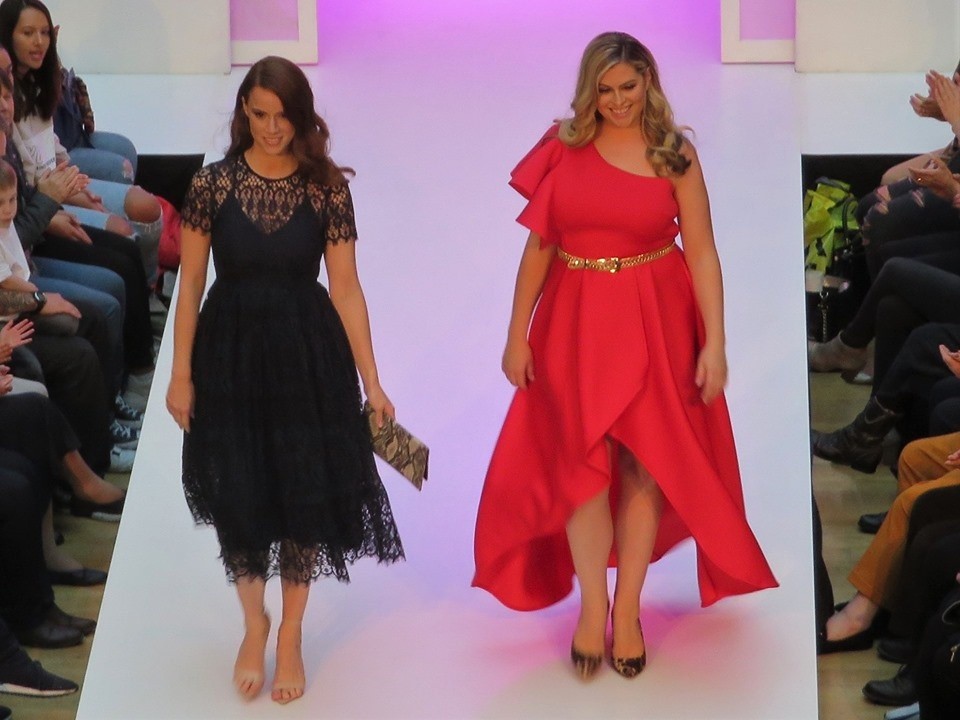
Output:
[272,624,305,705]
[234,615,271,701]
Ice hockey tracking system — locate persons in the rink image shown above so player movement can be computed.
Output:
[165,55,396,705]
[0,0,183,720]
[806,64,960,720]
[471,32,782,679]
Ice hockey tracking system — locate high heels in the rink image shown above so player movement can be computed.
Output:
[611,607,647,678]
[572,600,610,680]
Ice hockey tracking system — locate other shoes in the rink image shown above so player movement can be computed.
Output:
[807,176,948,717]
[0,169,181,719]
